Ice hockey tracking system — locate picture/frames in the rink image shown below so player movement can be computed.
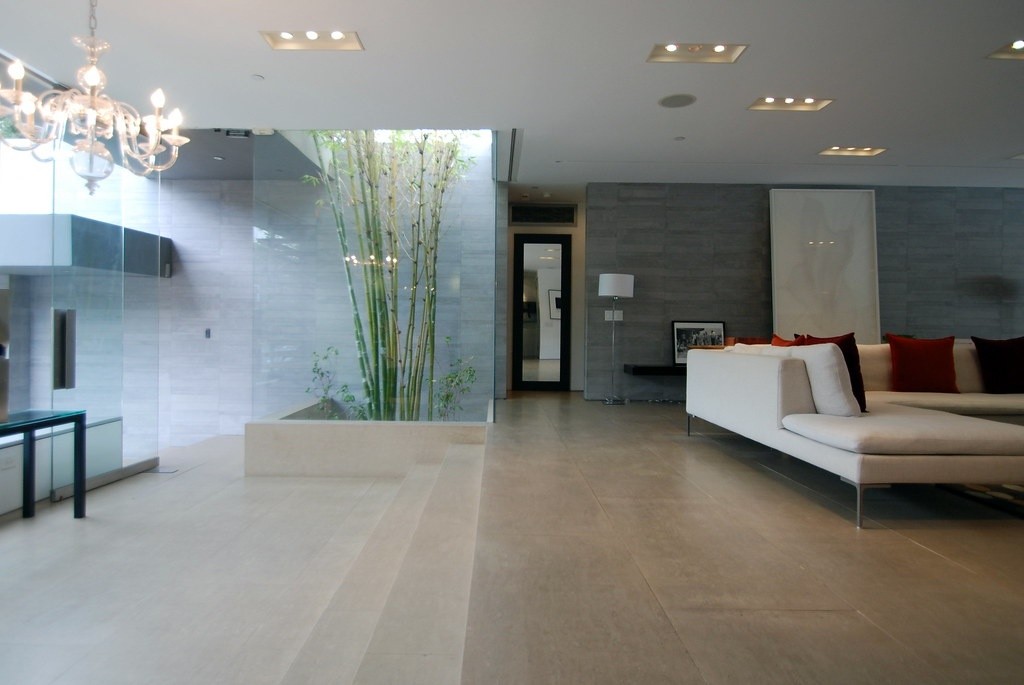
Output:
[672,318,726,369]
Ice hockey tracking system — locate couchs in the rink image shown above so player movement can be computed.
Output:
[683,335,1024,532]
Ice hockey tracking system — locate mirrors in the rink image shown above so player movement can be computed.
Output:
[510,232,573,394]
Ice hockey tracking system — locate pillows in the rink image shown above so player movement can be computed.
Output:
[803,332,871,416]
[972,333,1024,396]
[883,331,962,394]
[772,332,806,347]
[735,340,865,416]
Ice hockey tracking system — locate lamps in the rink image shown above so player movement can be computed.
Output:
[0,0,192,197]
[597,272,636,405]
